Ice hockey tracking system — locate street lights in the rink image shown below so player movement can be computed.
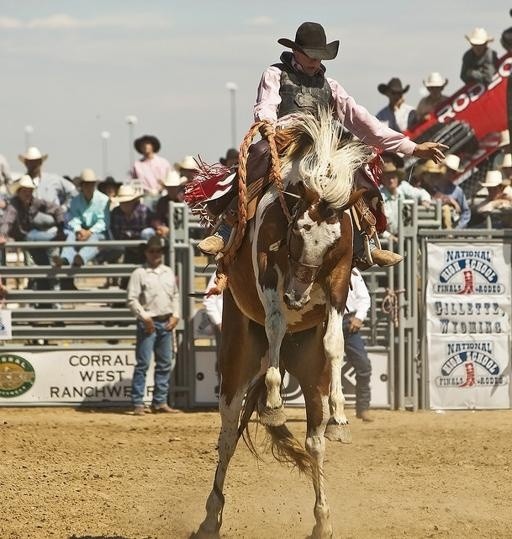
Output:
[122,107,139,166]
[22,122,34,153]
[97,129,109,182]
[224,81,241,156]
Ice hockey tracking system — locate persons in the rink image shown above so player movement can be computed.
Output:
[338,9,511,329]
[125,236,181,417]
[202,268,224,372]
[197,22,450,268]
[0,134,240,329]
[341,263,375,422]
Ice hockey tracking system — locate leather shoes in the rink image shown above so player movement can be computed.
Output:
[356,411,372,421]
[197,236,224,255]
[151,406,178,412]
[134,407,145,415]
[357,248,403,270]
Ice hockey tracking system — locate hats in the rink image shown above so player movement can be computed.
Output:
[382,152,405,179]
[278,22,339,60]
[466,27,493,46]
[496,129,512,168]
[74,169,144,202]
[220,149,239,165]
[158,156,198,186]
[423,72,447,88]
[10,175,39,194]
[479,171,510,187]
[18,147,48,162]
[140,236,167,252]
[419,154,464,174]
[135,135,160,152]
[378,78,408,94]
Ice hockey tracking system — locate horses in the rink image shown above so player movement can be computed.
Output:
[194,102,371,539]
